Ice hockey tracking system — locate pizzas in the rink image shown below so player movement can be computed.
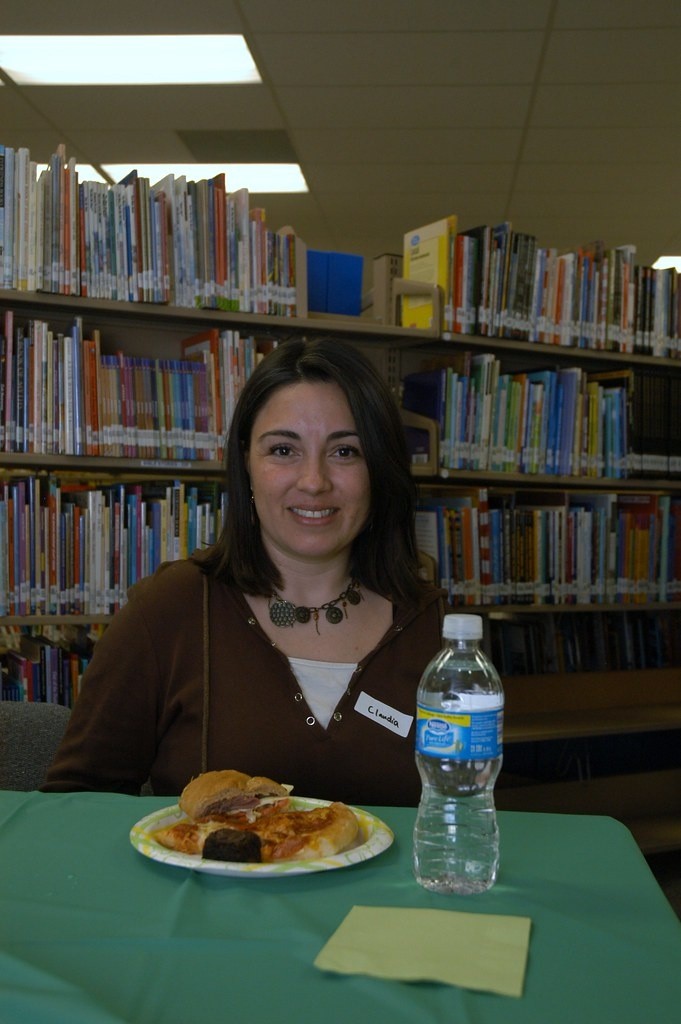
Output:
[157,799,358,864]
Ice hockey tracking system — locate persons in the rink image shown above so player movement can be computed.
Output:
[33,337,450,810]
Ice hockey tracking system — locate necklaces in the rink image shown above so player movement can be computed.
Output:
[268,571,367,635]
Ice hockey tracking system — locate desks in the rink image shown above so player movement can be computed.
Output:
[0,786,681,1023]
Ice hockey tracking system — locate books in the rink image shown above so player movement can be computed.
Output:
[1,481,681,617]
[0,307,681,480]
[0,138,681,358]
[0,609,681,713]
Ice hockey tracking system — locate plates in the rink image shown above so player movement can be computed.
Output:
[129,796,393,876]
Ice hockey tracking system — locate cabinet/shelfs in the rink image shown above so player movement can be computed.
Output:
[0,253,681,859]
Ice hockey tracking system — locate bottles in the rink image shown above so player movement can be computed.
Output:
[414,614,501,895]
